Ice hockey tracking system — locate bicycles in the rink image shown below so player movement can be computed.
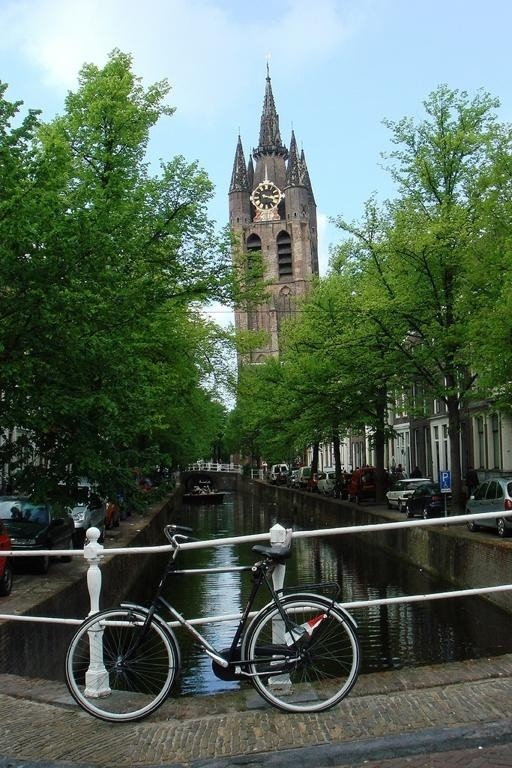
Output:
[64,523,362,722]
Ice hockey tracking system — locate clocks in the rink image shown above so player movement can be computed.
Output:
[248,179,286,213]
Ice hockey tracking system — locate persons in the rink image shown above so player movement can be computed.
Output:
[463,467,480,498]
[396,464,422,479]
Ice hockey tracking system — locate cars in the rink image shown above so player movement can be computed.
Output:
[268,464,512,537]
[0,467,169,597]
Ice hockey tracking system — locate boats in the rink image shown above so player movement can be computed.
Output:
[183,484,233,502]
